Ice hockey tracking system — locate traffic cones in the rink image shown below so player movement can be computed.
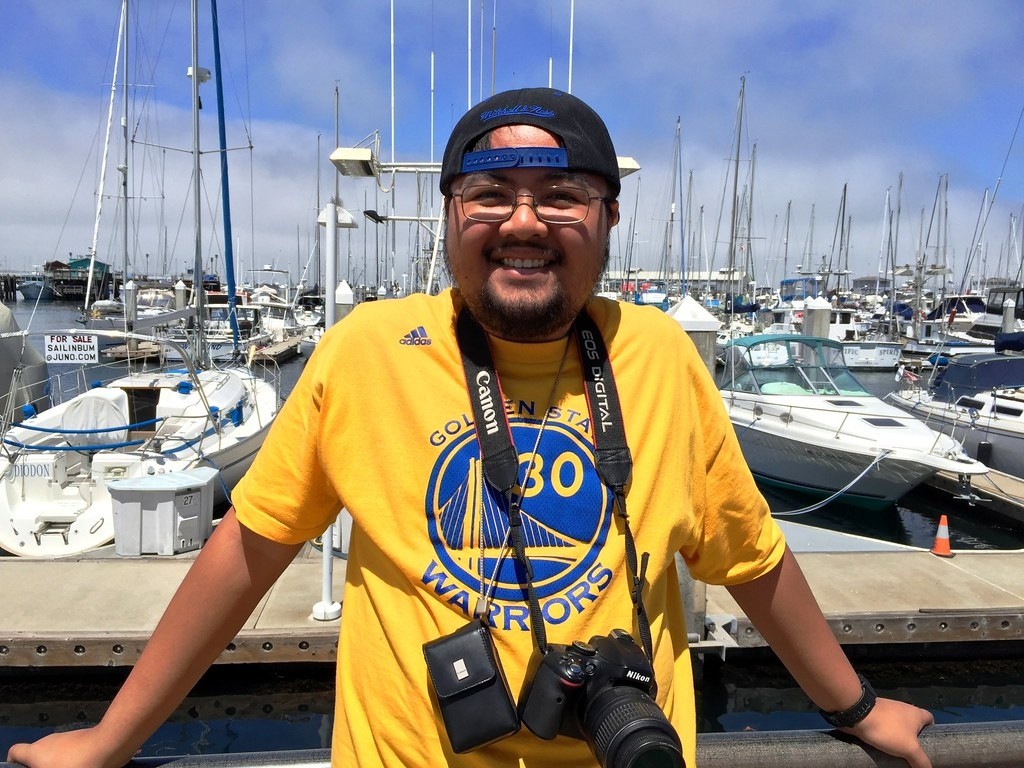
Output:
[926,514,957,559]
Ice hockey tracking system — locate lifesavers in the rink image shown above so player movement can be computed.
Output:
[795,312,804,319]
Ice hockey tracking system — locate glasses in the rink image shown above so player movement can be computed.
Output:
[449,184,611,224]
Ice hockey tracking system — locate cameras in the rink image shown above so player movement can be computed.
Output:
[513,630,686,768]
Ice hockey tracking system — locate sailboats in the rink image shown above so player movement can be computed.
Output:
[76,0,1024,369]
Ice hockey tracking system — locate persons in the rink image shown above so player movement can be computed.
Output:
[6,86,934,768]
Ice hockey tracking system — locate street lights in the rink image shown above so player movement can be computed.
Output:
[144,253,149,276]
[215,254,219,275]
[210,257,214,276]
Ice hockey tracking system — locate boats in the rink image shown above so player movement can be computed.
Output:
[1,1,288,564]
[883,351,1024,480]
[708,332,989,512]
[19,273,56,299]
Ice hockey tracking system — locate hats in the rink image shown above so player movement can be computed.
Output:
[438,87,621,225]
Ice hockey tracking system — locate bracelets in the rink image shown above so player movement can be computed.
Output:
[819,674,877,729]
